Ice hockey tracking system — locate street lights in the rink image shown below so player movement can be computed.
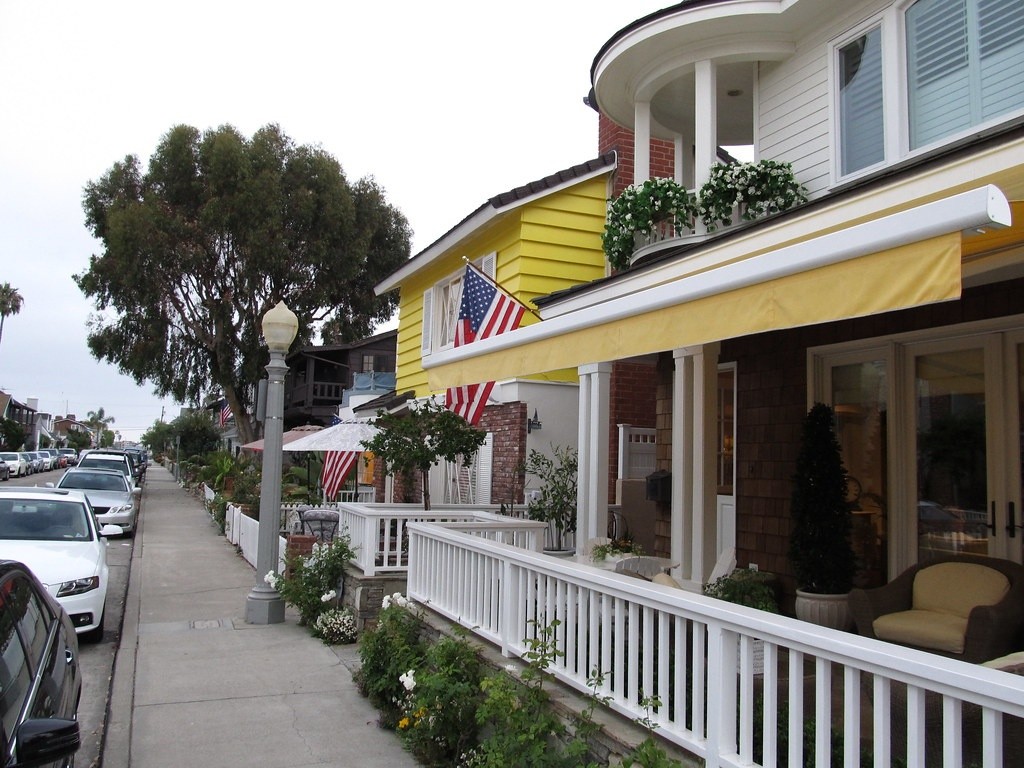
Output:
[243,299,300,624]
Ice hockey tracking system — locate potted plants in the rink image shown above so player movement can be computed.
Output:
[590,544,611,567]
[213,457,236,490]
[518,441,578,559]
[611,532,635,556]
[700,568,780,676]
[785,399,857,633]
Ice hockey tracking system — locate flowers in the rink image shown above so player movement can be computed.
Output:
[699,159,808,233]
[601,177,699,273]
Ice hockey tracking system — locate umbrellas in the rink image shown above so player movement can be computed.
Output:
[239,420,329,505]
[281,411,420,503]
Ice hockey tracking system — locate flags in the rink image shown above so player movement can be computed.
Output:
[321,414,361,501]
[219,398,232,427]
[443,262,527,431]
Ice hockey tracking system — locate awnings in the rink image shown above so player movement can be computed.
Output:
[421,184,1024,393]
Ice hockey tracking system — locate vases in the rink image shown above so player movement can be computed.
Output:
[379,541,396,564]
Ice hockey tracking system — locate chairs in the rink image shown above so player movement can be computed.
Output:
[615,556,681,583]
[303,510,339,551]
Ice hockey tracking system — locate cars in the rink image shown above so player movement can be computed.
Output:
[77,449,140,488]
[0,485,111,644]
[47,467,142,535]
[0,447,79,481]
[1,558,84,768]
[123,446,149,475]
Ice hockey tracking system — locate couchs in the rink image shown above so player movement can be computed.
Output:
[846,552,1024,666]
